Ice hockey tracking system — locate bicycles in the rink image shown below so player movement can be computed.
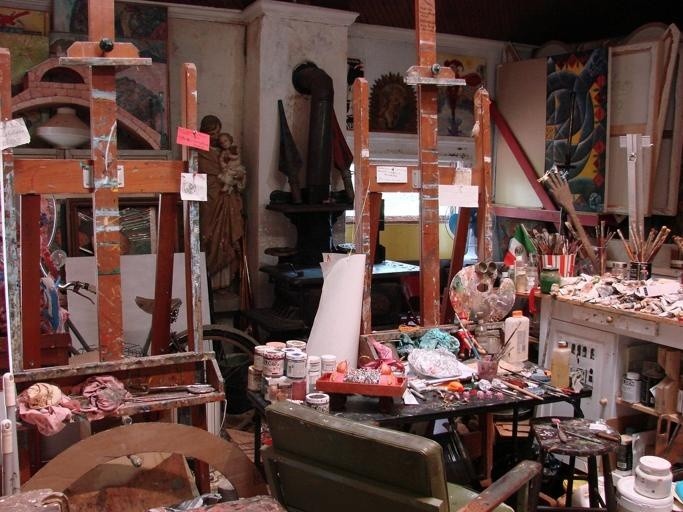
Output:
[39,258,270,434]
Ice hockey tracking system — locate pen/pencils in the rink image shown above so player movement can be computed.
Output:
[410,388,427,401]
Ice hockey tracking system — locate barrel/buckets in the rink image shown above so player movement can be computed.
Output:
[615,475,675,512]
[536,253,577,294]
[610,261,627,280]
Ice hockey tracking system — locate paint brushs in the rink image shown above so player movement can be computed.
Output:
[442,423,466,461]
[500,366,574,400]
[528,228,585,254]
[455,312,522,361]
[595,221,616,248]
[617,224,672,262]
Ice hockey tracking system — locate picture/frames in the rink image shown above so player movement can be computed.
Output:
[1,0,171,255]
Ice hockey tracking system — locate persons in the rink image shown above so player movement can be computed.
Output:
[219,132,241,195]
[197,114,246,292]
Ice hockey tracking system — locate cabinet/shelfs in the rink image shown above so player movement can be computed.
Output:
[534,299,683,478]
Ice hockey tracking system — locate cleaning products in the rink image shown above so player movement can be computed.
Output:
[551,340,572,389]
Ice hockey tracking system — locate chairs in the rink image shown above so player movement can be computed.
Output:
[260,399,542,512]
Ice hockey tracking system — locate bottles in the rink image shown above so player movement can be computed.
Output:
[612,262,627,280]
[632,456,673,499]
[548,340,571,389]
[473,312,487,355]
[540,268,561,294]
[514,255,526,293]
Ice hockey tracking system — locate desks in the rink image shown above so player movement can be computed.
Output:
[258,258,419,330]
[246,361,594,508]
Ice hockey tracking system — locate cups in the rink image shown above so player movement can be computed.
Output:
[476,356,498,383]
[541,253,575,277]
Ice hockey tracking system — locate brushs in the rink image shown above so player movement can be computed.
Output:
[552,418,568,443]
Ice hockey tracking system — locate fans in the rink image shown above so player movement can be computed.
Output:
[445,201,497,266]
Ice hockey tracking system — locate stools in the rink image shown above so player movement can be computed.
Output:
[518,416,622,512]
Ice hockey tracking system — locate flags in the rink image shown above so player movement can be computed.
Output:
[503,224,536,268]
